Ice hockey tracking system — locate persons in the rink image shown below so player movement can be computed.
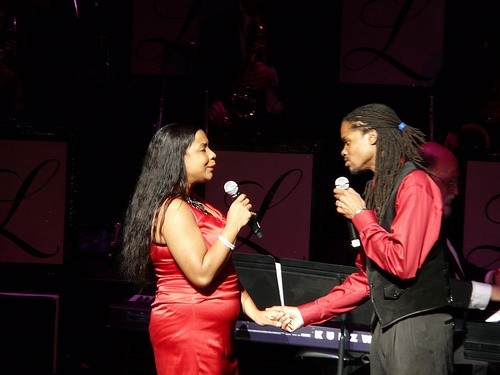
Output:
[122,123,295,375]
[413,141,500,364]
[267,104,457,375]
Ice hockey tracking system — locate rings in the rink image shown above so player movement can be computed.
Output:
[335,200,340,205]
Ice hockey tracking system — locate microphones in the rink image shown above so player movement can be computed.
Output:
[335,177,360,247]
[224,181,263,239]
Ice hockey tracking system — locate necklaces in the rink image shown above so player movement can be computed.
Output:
[185,197,206,213]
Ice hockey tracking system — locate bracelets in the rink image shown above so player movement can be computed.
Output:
[218,235,235,251]
[354,207,366,215]
